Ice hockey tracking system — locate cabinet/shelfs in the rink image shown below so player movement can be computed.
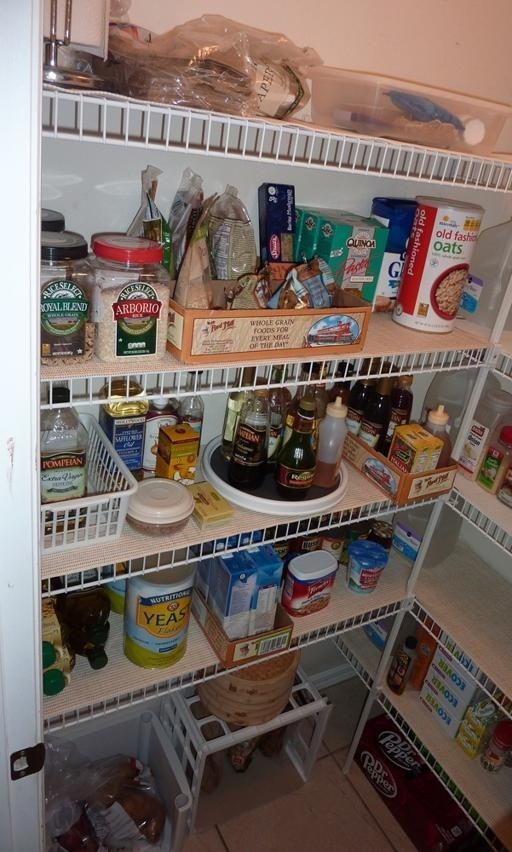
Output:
[328,273,512,848]
[42,78,510,780]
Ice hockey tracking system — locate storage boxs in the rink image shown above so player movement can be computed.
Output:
[40,409,136,555]
[42,707,194,852]
[159,661,334,809]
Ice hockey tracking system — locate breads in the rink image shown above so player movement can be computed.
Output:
[52,753,165,849]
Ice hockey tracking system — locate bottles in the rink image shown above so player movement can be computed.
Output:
[41,208,97,369]
[387,635,418,697]
[156,423,201,485]
[101,547,199,670]
[314,396,350,488]
[41,569,113,697]
[282,550,338,618]
[276,395,319,496]
[220,363,328,483]
[141,387,180,473]
[337,357,414,458]
[457,388,512,509]
[90,231,166,365]
[39,387,89,533]
[178,371,205,457]
[425,404,452,469]
[390,194,484,335]
[270,507,394,567]
[479,719,512,774]
[98,379,150,482]
